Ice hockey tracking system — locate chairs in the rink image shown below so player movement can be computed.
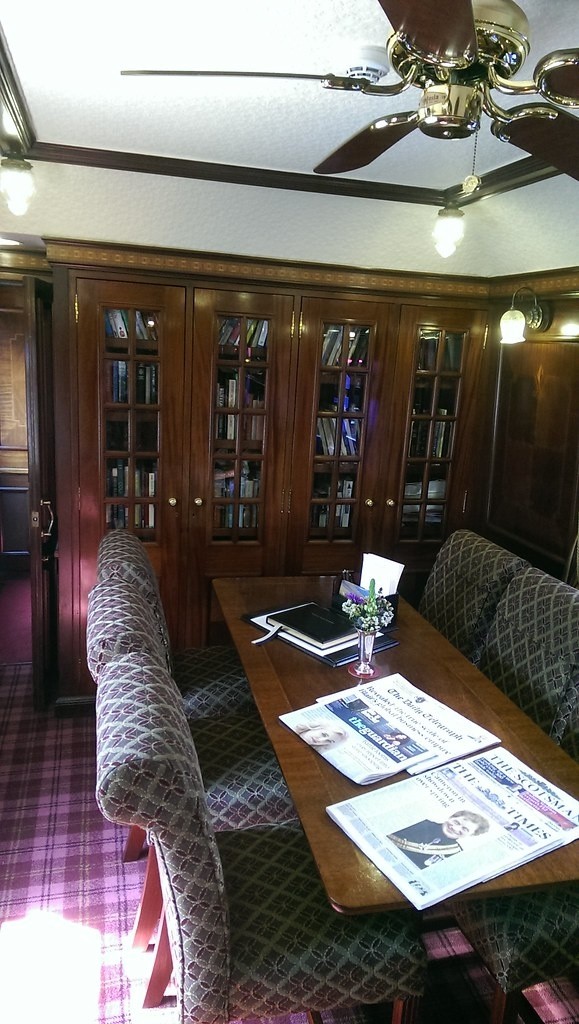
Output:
[97,528,257,715]
[461,566,579,743]
[435,667,579,1024]
[83,577,301,951]
[94,649,432,1024]
[418,528,532,665]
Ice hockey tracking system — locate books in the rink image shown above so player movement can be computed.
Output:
[218,317,268,360]
[110,421,156,450]
[327,371,364,413]
[215,371,265,440]
[106,458,156,529]
[409,404,448,465]
[106,360,158,405]
[240,601,400,667]
[325,747,579,910]
[404,479,446,523]
[104,308,159,340]
[320,323,369,367]
[213,449,260,528]
[311,476,353,528]
[316,416,361,465]
[418,333,461,371]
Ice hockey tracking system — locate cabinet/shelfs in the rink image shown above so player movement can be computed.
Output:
[50,264,511,724]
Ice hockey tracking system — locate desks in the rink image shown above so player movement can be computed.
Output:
[212,574,579,918]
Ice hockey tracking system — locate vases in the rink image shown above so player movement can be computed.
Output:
[352,623,382,676]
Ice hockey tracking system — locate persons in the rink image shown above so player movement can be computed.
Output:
[296,723,348,752]
[388,811,489,870]
[383,733,410,746]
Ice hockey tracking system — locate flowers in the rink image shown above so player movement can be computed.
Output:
[341,578,395,631]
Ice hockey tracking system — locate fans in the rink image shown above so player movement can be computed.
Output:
[115,0,579,181]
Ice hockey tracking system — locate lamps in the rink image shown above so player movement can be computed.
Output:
[498,284,553,348]
[0,157,39,217]
[423,205,471,262]
